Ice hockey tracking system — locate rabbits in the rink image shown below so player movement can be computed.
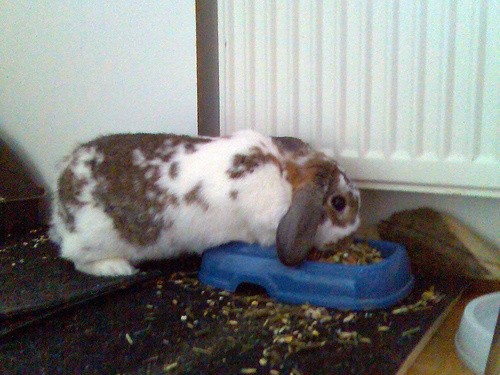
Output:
[44,131,362,280]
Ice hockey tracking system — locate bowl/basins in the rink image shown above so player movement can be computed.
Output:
[454,290,500,375]
